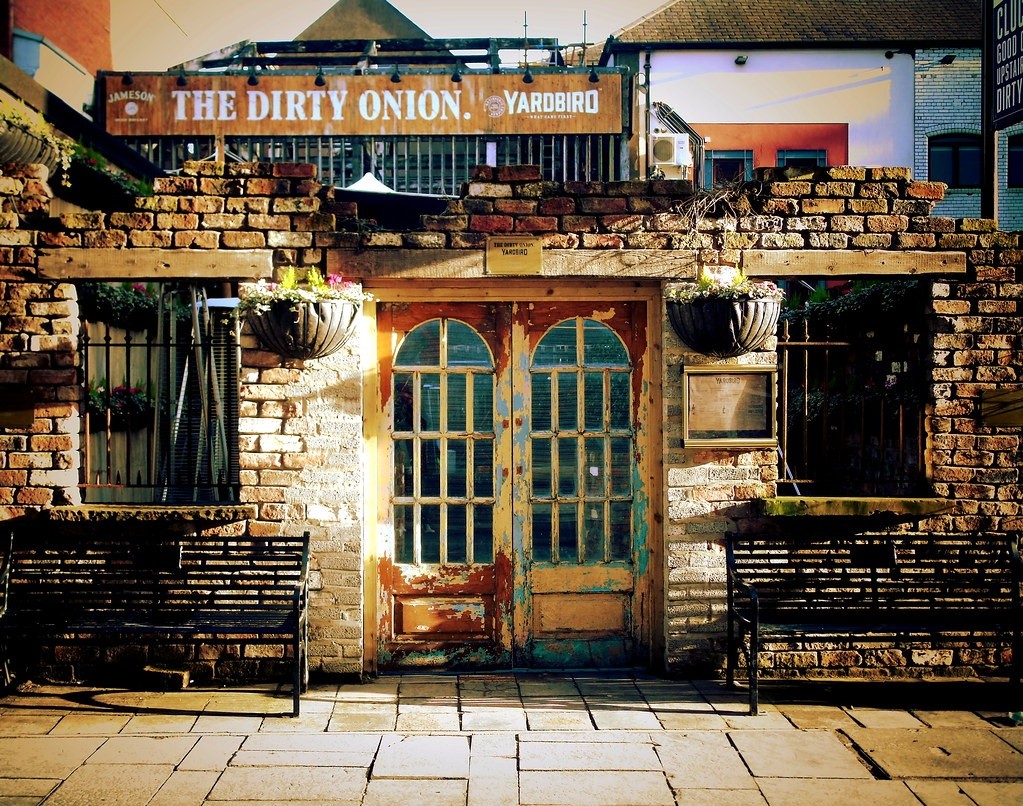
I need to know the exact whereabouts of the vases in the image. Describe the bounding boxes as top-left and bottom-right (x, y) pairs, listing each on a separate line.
(666, 295), (782, 357)
(89, 409), (154, 433)
(246, 300), (362, 358)
(87, 307), (192, 334)
(48, 158), (137, 214)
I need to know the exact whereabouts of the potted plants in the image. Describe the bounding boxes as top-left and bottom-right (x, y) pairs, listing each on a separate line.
(0, 98), (75, 188)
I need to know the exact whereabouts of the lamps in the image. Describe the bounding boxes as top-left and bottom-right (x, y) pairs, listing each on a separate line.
(315, 62), (326, 86)
(523, 64), (533, 83)
(177, 67), (186, 86)
(941, 55), (956, 64)
(451, 63), (462, 82)
(391, 62), (401, 82)
(248, 63), (259, 85)
(122, 71), (133, 84)
(636, 73), (649, 94)
(735, 56), (749, 64)
(589, 62), (600, 82)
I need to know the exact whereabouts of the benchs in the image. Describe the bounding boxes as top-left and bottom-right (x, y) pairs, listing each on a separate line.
(0, 531), (310, 718)
(724, 529), (1023, 717)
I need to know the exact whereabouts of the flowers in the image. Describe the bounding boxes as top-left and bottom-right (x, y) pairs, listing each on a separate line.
(87, 374), (159, 413)
(220, 264), (374, 337)
(664, 260), (786, 303)
(71, 135), (154, 197)
(79, 280), (203, 322)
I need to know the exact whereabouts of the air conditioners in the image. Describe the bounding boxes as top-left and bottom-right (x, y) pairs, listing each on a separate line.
(651, 133), (690, 166)
